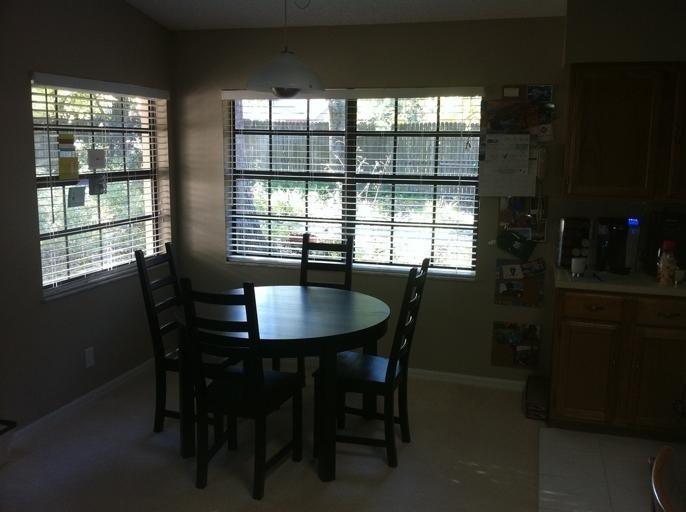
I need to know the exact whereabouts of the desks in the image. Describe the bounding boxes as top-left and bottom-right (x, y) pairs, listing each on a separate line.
(179, 285), (390, 482)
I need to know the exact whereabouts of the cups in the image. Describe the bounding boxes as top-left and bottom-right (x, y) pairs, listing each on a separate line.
(87, 148), (108, 170)
(59, 156), (80, 181)
(571, 256), (587, 283)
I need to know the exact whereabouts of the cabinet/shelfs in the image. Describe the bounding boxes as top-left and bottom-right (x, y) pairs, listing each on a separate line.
(549, 290), (686, 442)
(564, 60), (685, 205)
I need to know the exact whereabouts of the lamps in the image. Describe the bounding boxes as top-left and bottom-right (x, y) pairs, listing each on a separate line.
(247, 0), (326, 98)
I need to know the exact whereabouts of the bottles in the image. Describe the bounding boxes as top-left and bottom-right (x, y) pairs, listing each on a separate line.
(656, 239), (677, 285)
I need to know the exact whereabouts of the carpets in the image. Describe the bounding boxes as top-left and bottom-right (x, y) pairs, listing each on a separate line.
(1, 357), (546, 512)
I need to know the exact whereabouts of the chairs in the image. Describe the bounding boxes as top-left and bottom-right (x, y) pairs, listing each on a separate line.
(648, 445), (678, 512)
(176, 279), (301, 500)
(273, 233), (353, 386)
(313, 259), (429, 466)
(134, 243), (241, 443)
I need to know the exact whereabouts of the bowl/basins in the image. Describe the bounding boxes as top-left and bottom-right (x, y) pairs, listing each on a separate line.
(673, 269), (686, 283)
